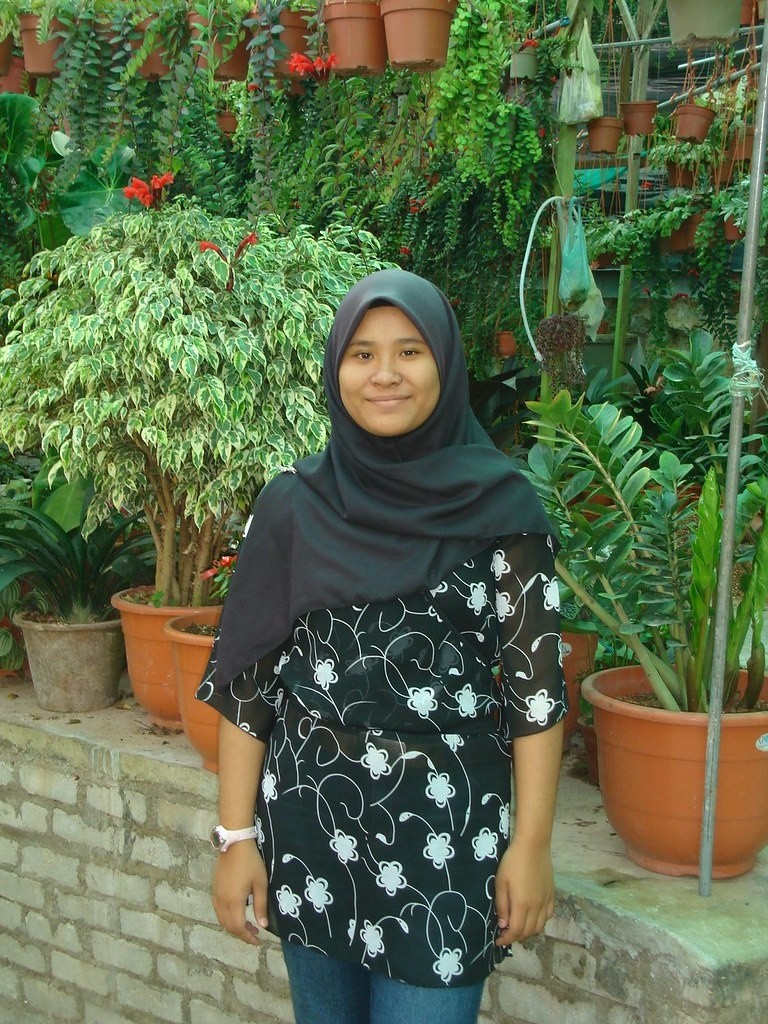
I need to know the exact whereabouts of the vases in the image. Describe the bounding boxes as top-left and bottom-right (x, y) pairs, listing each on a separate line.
(587, 117), (623, 155)
(159, 605), (223, 778)
(742, 0), (759, 27)
(666, 0), (743, 51)
(619, 100), (660, 134)
(674, 103), (717, 142)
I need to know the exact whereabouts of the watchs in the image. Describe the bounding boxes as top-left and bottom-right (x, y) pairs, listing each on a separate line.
(209, 824), (257, 852)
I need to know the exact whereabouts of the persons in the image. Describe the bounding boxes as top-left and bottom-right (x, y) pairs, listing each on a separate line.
(190, 267), (570, 1024)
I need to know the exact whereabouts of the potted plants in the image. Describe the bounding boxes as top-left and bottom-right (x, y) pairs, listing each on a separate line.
(0, 443), (181, 712)
(0, 192), (404, 732)
(493, 173), (768, 876)
(510, 33), (583, 105)
(705, 113), (734, 184)
(645, 140), (715, 188)
(0, 0), (460, 138)
(712, 73), (759, 161)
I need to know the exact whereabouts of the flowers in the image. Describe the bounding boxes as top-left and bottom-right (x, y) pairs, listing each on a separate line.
(191, 516), (251, 601)
